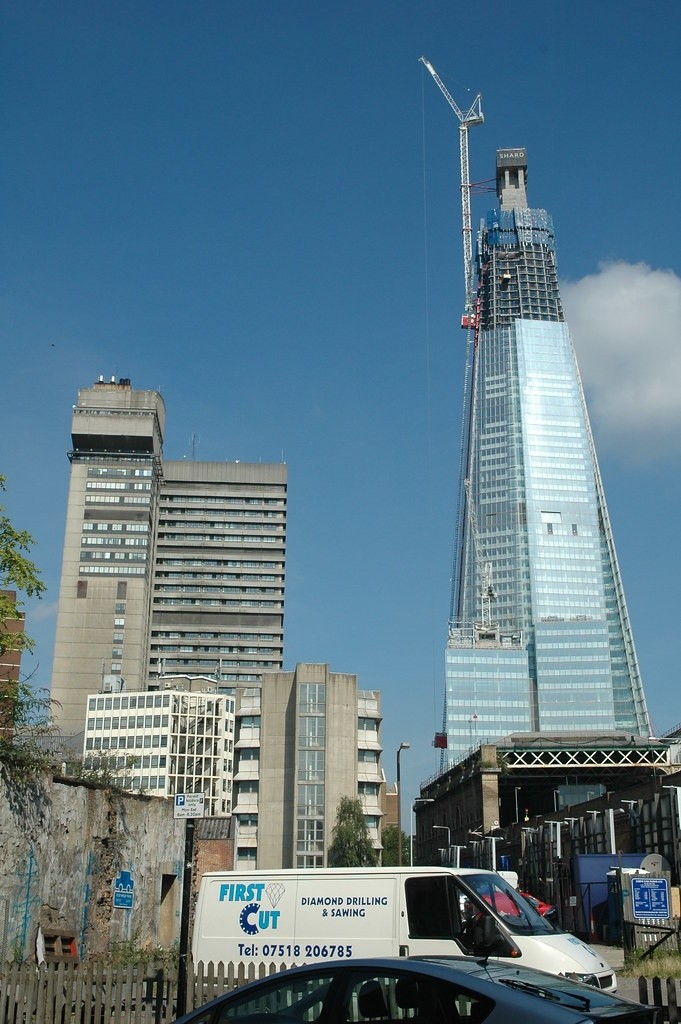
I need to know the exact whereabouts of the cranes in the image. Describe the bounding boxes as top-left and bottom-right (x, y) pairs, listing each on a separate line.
(460, 475), (501, 632)
(417, 52), (488, 331)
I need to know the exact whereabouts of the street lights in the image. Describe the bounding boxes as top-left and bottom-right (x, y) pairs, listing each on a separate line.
(553, 789), (560, 813)
(515, 786), (522, 824)
(396, 743), (411, 867)
(432, 825), (451, 866)
(588, 792), (594, 801)
(409, 799), (436, 867)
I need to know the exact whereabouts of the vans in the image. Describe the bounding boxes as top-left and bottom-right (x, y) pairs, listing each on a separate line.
(192, 866), (616, 998)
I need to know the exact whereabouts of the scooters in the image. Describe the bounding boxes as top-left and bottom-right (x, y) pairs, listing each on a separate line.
(459, 898), (557, 956)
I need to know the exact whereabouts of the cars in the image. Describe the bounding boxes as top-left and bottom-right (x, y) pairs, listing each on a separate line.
(167, 955), (680, 1024)
